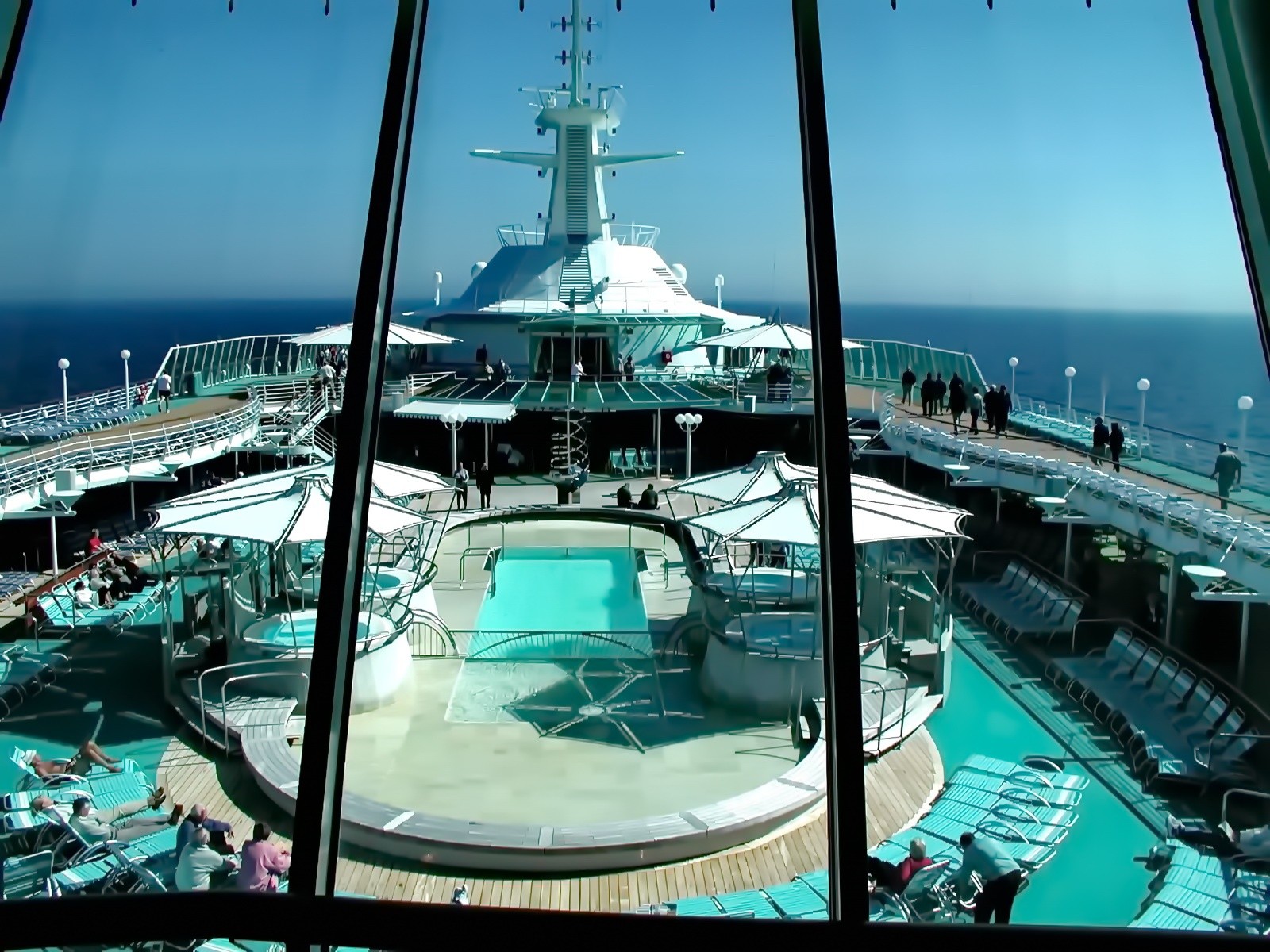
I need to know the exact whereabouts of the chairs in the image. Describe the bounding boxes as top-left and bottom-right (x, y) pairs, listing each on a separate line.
(610, 413), (1270, 935)
(0, 407), (374, 952)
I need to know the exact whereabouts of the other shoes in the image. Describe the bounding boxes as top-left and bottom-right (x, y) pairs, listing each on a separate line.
(458, 884), (471, 906)
(153, 786), (163, 798)
(452, 887), (462, 902)
(152, 795), (166, 811)
(168, 805), (183, 825)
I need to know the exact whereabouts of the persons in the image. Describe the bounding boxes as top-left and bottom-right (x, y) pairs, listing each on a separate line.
(21, 469), (290, 891)
(901, 366), (917, 407)
(1052, 550), (1074, 580)
(1108, 423), (1124, 472)
(159, 346), (791, 415)
(867, 814), (1270, 929)
(921, 373), (1012, 438)
(1091, 416), (1109, 467)
(454, 461), (789, 569)
(1078, 548), (1100, 620)
(1210, 443), (1242, 511)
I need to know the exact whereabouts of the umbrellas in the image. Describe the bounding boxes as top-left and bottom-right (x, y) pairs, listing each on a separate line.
(687, 321), (869, 381)
(282, 319), (463, 373)
(659, 448), (973, 655)
(144, 458), (465, 665)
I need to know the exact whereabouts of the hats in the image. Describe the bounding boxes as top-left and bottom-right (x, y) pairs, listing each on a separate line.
(21, 749), (36, 765)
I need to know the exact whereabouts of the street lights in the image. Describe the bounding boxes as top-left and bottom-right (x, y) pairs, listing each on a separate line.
(1136, 378), (1151, 461)
(1008, 357), (1019, 395)
(1237, 395), (1254, 462)
(57, 358), (70, 420)
(676, 412), (703, 481)
(121, 349), (132, 410)
(1065, 366), (1075, 421)
